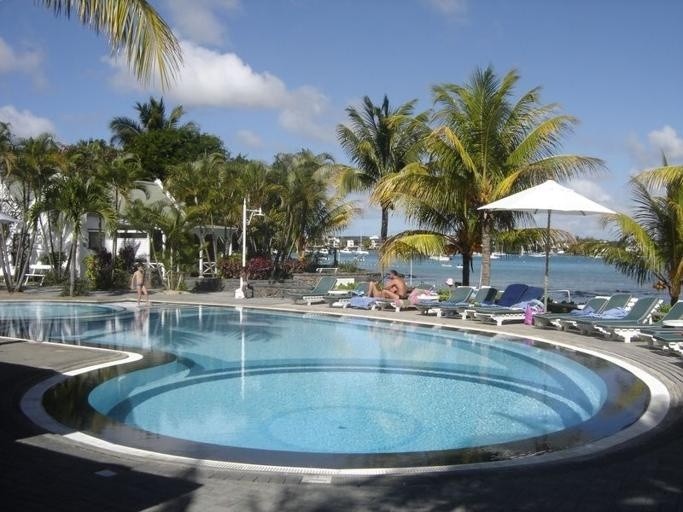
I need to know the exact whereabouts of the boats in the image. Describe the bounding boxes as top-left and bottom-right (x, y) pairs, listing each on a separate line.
(306, 238), (549, 270)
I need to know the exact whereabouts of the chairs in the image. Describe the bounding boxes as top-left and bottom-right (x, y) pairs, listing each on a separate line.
(532, 293), (683, 357)
(286, 276), (430, 312)
(413, 284), (545, 327)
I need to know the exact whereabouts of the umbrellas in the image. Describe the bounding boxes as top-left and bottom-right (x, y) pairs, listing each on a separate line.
(475, 179), (622, 314)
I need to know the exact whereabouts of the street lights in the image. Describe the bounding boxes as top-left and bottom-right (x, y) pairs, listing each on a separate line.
(234, 198), (265, 301)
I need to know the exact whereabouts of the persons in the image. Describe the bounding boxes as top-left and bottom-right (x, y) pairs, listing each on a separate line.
(129, 262), (149, 303)
(132, 306), (149, 340)
(380, 269), (408, 299)
(366, 280), (397, 298)
(238, 266), (255, 298)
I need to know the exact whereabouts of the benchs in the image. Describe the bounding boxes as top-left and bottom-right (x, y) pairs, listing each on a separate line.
(22, 264), (54, 287)
